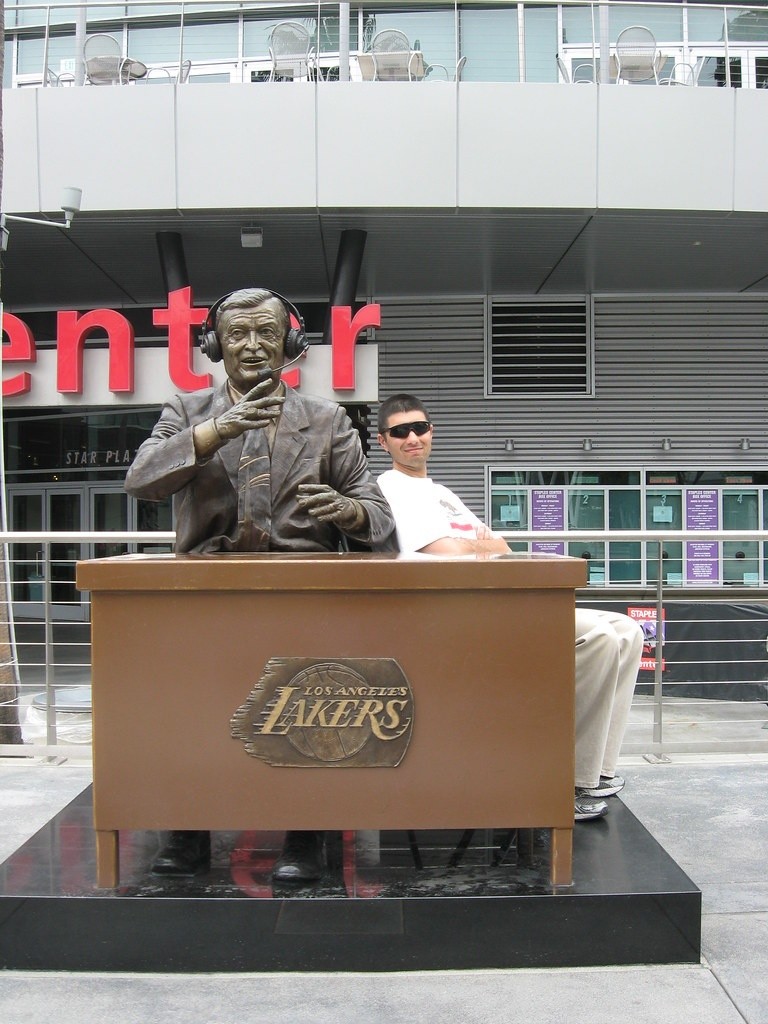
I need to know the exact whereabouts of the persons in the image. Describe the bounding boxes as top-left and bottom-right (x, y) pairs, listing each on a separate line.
(123, 287), (396, 882)
(375, 394), (643, 820)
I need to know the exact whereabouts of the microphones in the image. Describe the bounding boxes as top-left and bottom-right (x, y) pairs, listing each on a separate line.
(256, 349), (306, 380)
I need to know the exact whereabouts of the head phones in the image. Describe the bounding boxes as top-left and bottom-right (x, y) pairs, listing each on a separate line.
(198, 287), (309, 363)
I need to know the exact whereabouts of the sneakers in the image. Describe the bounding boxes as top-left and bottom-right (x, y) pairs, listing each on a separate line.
(580, 776), (625, 797)
(574, 787), (608, 820)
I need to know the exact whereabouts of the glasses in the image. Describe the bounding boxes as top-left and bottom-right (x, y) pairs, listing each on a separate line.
(380, 421), (431, 438)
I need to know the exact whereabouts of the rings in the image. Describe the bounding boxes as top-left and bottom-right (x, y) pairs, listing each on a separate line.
(248, 407), (259, 417)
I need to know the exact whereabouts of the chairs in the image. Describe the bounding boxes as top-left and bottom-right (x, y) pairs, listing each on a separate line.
(46, 21), (706, 87)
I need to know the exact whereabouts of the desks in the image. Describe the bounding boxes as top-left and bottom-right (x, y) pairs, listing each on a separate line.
(74, 552), (591, 887)
(609, 53), (667, 85)
(81, 55), (147, 85)
(356, 51), (424, 81)
